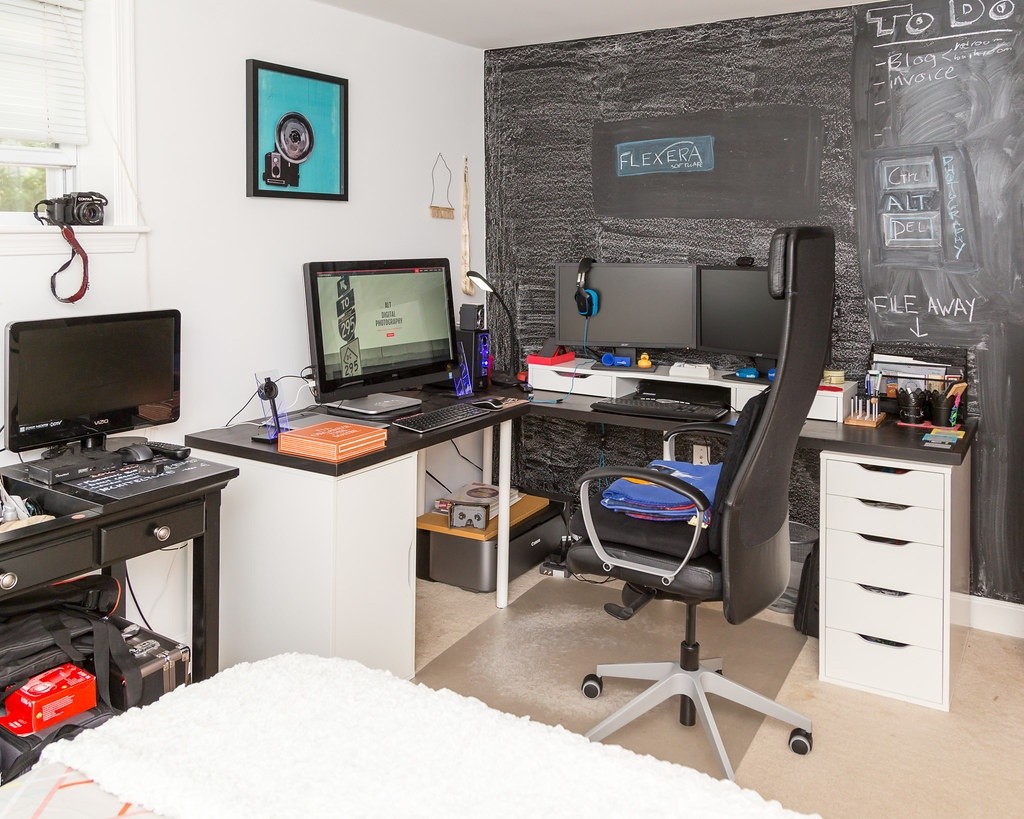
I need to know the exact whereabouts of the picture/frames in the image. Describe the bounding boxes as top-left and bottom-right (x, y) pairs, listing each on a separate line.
(247, 58), (351, 200)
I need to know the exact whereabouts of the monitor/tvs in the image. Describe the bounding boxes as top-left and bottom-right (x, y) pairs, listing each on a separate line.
(696, 265), (786, 386)
(3, 308), (181, 455)
(554, 262), (696, 373)
(303, 257), (458, 420)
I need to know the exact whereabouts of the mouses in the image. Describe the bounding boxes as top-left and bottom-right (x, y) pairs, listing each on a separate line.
(472, 398), (503, 410)
(114, 444), (153, 464)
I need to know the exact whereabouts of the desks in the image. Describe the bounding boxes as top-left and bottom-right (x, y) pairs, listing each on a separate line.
(0, 434), (243, 680)
(186, 371), (989, 502)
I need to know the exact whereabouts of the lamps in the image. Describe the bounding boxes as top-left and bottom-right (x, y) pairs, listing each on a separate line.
(467, 269), (526, 388)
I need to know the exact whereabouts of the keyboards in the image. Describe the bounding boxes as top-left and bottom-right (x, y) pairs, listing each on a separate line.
(590, 397), (729, 422)
(391, 402), (490, 433)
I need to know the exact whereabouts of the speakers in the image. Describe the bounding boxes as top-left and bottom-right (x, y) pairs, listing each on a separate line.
(459, 303), (484, 331)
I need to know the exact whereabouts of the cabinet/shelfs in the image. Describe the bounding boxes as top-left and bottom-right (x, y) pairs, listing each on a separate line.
(816, 449), (977, 711)
(179, 446), (422, 681)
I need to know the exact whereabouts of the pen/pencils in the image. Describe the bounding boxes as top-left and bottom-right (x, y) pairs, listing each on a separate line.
(864, 372), (871, 395)
(875, 370), (883, 395)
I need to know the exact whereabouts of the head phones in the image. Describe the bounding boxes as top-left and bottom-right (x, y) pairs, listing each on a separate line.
(574, 258), (599, 317)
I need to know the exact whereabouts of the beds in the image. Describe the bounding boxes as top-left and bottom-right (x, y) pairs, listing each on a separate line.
(1, 652), (821, 817)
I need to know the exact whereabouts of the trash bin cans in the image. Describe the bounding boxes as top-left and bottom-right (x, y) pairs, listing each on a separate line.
(766, 520), (819, 616)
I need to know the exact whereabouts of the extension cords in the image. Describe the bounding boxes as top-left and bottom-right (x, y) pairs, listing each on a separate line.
(539, 560), (572, 578)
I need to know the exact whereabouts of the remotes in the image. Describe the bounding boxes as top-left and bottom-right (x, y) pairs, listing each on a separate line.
(141, 441), (191, 460)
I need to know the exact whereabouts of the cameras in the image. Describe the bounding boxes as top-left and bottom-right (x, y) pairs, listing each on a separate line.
(34, 191), (108, 226)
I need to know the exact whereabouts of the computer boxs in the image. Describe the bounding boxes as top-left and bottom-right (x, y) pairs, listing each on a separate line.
(422, 326), (490, 393)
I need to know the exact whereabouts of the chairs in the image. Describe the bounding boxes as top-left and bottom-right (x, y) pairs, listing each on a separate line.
(564, 224), (837, 783)
